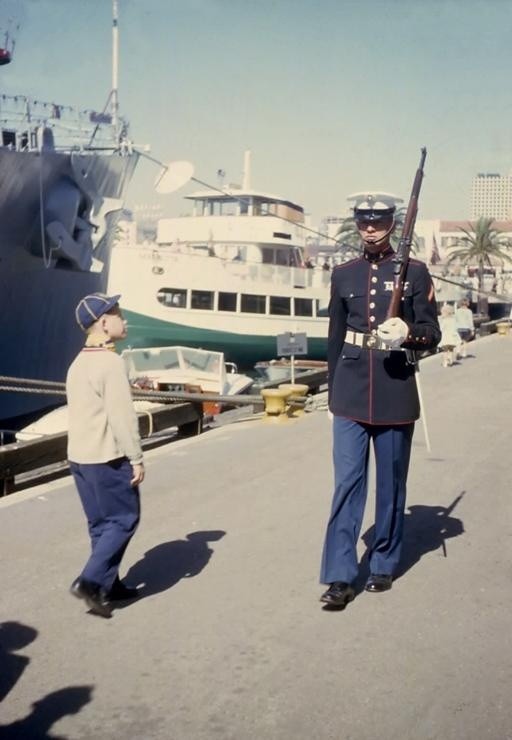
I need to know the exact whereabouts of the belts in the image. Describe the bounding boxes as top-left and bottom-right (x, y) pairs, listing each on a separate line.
(344, 330), (406, 351)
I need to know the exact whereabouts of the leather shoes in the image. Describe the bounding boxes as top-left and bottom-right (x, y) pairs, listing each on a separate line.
(72, 576), (137, 618)
(320, 581), (355, 605)
(366, 573), (392, 591)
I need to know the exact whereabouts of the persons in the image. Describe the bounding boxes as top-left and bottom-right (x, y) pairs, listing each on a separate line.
(454, 298), (475, 359)
(317, 191), (444, 608)
(64, 291), (146, 620)
(437, 304), (462, 367)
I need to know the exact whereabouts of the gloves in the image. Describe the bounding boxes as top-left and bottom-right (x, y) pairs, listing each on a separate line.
(377, 318), (409, 346)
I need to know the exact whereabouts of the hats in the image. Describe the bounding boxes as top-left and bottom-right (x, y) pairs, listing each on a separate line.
(75, 293), (120, 331)
(354, 199), (396, 221)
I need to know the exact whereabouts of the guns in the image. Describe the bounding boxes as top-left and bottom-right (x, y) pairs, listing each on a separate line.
(386, 147), (426, 320)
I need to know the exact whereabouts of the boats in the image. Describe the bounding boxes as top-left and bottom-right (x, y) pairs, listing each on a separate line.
(1, 1), (140, 447)
(14, 345), (254, 445)
(427, 270), (511, 339)
(254, 357), (328, 382)
(108, 150), (330, 393)
